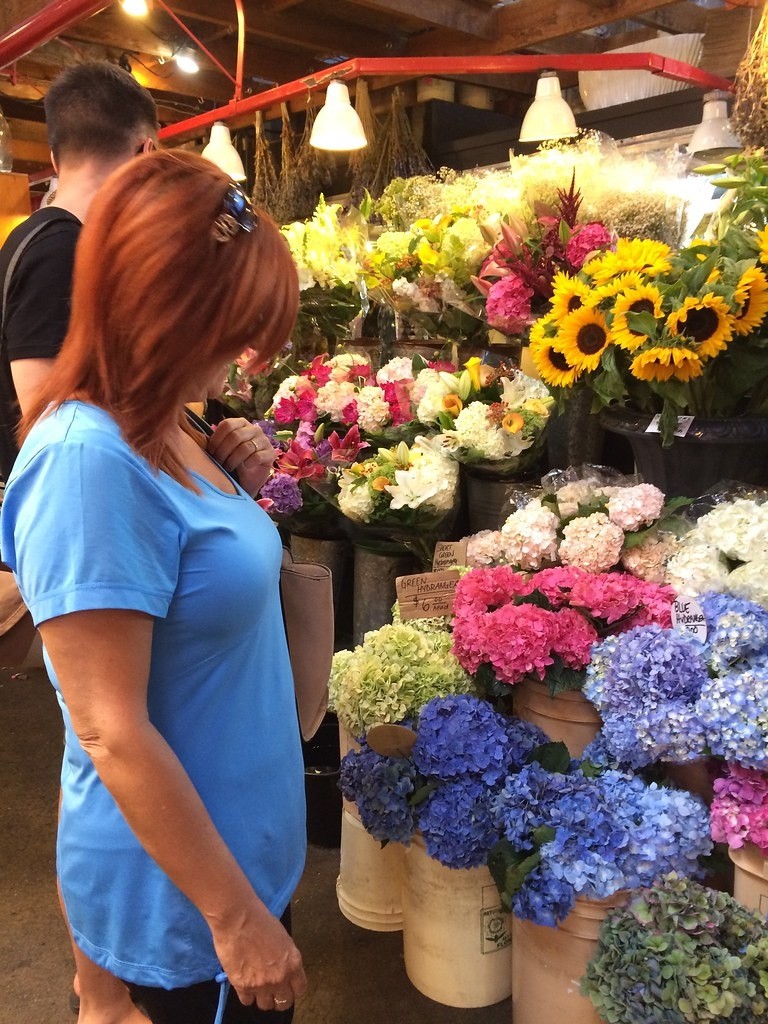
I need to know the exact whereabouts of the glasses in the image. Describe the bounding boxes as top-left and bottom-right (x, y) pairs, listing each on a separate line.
(210, 180), (261, 248)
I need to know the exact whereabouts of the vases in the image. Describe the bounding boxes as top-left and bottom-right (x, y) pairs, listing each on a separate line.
(346, 333), (386, 369)
(727, 839), (768, 915)
(666, 750), (723, 804)
(334, 805), (402, 935)
(337, 700), (380, 824)
(390, 338), (455, 363)
(347, 537), (417, 651)
(504, 883), (643, 1024)
(399, 827), (511, 1009)
(465, 455), (519, 532)
(597, 397), (768, 497)
(291, 525), (343, 581)
(508, 677), (600, 762)
(458, 346), (509, 370)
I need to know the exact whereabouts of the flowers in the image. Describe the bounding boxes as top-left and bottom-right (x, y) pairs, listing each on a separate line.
(213, 127), (768, 1024)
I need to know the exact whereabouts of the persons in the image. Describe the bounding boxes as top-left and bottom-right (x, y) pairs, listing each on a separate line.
(0, 148), (310, 1024)
(0, 59), (207, 1024)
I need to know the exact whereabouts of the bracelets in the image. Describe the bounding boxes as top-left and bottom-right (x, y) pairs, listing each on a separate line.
(203, 400), (208, 413)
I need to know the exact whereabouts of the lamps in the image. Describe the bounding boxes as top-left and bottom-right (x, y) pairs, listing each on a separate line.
(175, 39), (202, 75)
(202, 116), (249, 186)
(516, 68), (580, 144)
(305, 81), (370, 156)
(685, 87), (747, 162)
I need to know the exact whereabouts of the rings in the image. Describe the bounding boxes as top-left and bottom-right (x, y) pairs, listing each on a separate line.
(275, 997), (287, 1004)
(251, 440), (258, 451)
